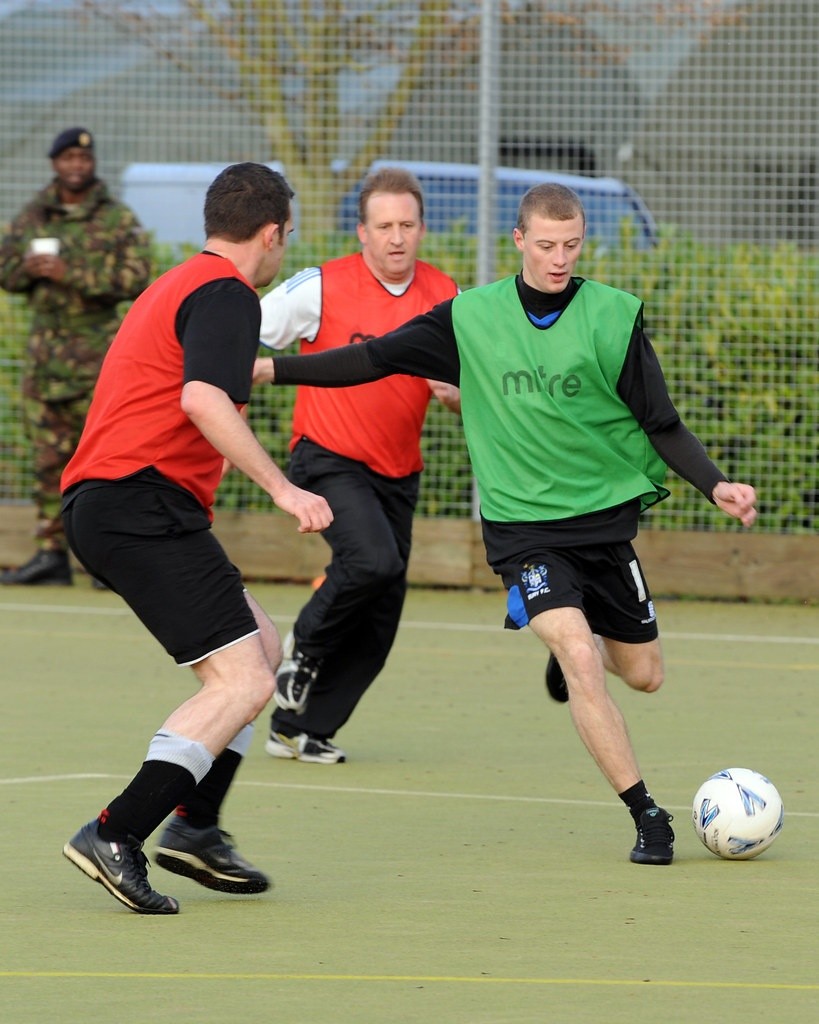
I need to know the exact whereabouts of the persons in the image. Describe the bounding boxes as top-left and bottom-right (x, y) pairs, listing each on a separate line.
(60, 162), (335, 912)
(0, 128), (151, 594)
(252, 181), (759, 863)
(260, 170), (461, 765)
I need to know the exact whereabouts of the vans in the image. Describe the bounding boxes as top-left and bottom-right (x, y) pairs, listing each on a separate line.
(120, 158), (659, 254)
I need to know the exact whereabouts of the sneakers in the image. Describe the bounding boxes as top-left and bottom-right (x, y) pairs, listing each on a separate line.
(62, 818), (178, 914)
(546, 651), (571, 702)
(266, 726), (345, 764)
(155, 815), (269, 894)
(629, 808), (676, 863)
(272, 628), (319, 710)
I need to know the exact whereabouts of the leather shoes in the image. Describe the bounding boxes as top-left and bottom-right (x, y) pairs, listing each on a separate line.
(0, 548), (73, 587)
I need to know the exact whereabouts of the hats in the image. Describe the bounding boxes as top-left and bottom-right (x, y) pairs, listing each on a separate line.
(49, 127), (92, 159)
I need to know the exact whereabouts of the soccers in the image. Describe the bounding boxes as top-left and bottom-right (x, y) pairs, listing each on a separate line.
(691, 767), (785, 862)
